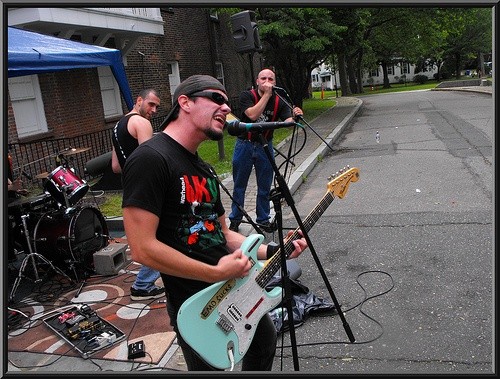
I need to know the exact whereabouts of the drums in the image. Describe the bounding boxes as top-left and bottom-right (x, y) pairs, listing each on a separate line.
(42, 164), (90, 207)
(30, 203), (110, 270)
(31, 191), (57, 211)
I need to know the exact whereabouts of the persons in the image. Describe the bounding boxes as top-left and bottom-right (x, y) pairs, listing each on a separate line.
(229, 68), (302, 233)
(112, 88), (165, 301)
(121, 75), (277, 371)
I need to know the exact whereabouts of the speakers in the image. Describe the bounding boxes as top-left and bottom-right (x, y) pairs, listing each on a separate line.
(230, 10), (262, 52)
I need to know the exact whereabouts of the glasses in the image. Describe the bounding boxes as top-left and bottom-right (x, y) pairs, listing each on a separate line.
(188, 91), (229, 106)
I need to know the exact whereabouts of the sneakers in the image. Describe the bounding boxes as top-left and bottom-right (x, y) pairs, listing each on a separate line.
(130, 285), (166, 301)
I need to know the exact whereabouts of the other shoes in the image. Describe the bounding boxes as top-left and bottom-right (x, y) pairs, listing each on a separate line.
(261, 223), (272, 233)
(229, 223), (239, 232)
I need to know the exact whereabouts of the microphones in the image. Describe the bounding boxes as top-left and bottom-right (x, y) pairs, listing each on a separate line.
(271, 85), (285, 91)
(228, 121), (296, 136)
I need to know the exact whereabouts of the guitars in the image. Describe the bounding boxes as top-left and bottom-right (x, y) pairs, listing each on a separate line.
(176, 164), (359, 369)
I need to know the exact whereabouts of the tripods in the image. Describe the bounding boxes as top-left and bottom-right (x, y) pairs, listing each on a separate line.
(6, 206), (73, 303)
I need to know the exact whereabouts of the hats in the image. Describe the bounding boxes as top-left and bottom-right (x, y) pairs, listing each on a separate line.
(158, 74), (227, 130)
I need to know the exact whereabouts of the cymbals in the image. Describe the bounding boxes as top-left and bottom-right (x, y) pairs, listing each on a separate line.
(35, 167), (75, 179)
(7, 193), (47, 207)
(50, 146), (92, 158)
